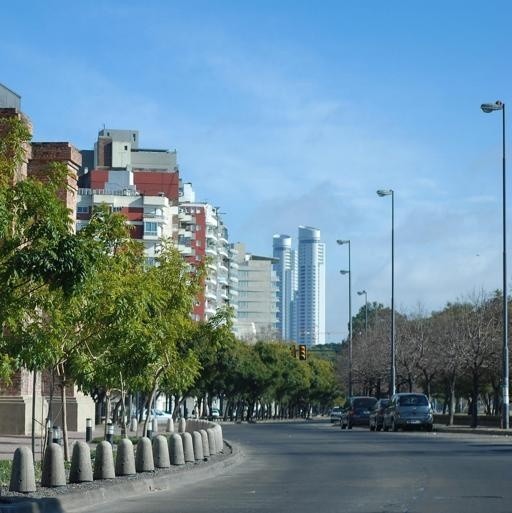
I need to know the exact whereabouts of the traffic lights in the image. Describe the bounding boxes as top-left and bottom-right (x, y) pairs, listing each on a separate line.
(299, 344), (307, 360)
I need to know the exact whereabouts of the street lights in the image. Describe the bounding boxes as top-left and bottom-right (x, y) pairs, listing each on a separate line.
(339, 269), (351, 274)
(375, 188), (396, 398)
(335, 239), (353, 398)
(481, 97), (512, 428)
(355, 289), (368, 343)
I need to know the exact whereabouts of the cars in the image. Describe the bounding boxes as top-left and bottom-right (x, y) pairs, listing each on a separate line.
(126, 405), (269, 421)
(330, 392), (433, 431)
